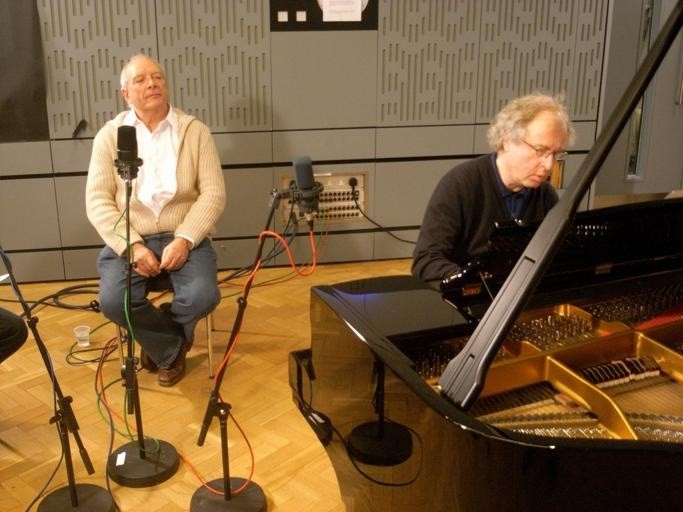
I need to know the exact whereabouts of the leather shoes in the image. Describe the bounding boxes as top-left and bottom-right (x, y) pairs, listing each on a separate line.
(141, 346), (157, 370)
(158, 334), (195, 387)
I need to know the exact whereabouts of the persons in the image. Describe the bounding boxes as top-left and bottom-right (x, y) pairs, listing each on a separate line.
(411, 94), (576, 282)
(86, 54), (226, 387)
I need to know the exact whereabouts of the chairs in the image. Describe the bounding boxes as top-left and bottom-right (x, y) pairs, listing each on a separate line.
(117, 270), (216, 379)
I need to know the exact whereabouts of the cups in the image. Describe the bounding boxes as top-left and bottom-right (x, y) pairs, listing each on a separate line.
(73, 325), (90, 347)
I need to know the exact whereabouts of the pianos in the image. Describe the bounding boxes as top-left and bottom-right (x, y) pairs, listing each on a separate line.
(289, 194), (681, 512)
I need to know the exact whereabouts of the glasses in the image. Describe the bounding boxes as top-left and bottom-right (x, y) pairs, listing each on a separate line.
(520, 138), (568, 161)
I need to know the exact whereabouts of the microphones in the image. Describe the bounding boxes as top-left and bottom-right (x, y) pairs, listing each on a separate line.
(292, 156), (320, 231)
(114, 125), (144, 197)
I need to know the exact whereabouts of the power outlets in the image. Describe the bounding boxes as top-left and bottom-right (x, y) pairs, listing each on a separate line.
(283, 174), (363, 191)
